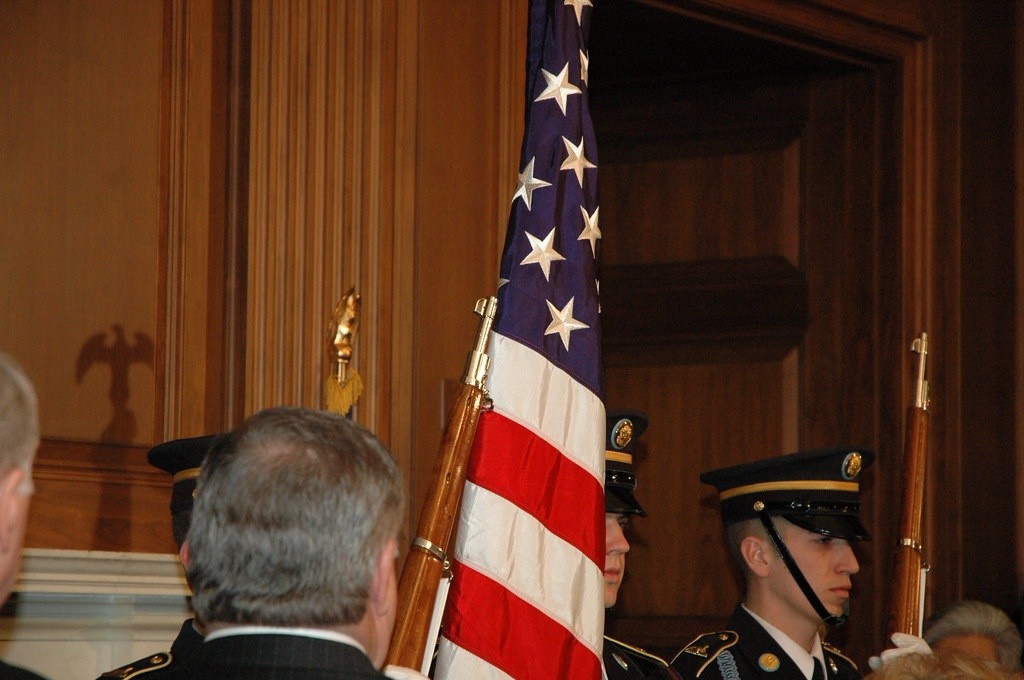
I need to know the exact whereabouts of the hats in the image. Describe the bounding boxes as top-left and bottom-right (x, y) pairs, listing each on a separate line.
(699, 446), (874, 541)
(604, 410), (647, 516)
(146, 433), (225, 510)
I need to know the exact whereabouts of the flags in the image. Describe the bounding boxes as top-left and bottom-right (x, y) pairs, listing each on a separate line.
(431, 1), (607, 680)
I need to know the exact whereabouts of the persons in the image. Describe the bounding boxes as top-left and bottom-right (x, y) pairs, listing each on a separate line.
(180, 406), (405, 680)
(926, 600), (1024, 672)
(97, 433), (215, 680)
(669, 445), (933, 680)
(0, 352), (49, 680)
(866, 652), (1020, 680)
(606, 412), (680, 680)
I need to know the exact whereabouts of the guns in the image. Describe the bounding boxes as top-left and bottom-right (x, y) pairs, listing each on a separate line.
(377, 293), (501, 679)
(889, 331), (931, 648)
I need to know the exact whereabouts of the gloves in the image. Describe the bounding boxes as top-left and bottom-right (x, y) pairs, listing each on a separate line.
(868, 633), (933, 671)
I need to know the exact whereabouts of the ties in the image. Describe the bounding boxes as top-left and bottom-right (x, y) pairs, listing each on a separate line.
(811, 657), (824, 680)
